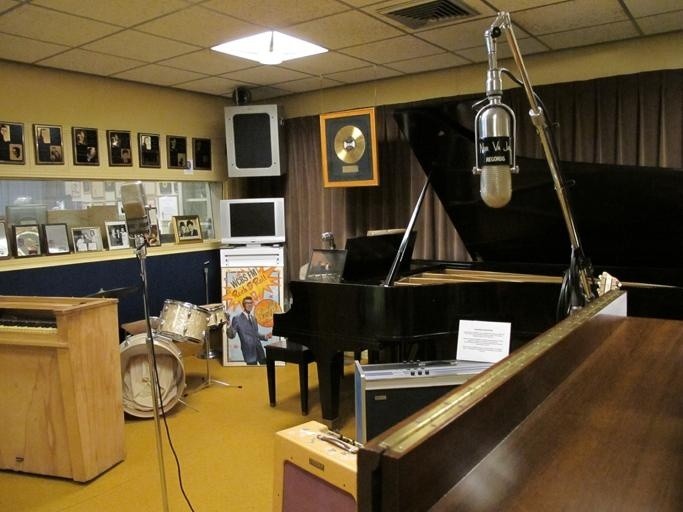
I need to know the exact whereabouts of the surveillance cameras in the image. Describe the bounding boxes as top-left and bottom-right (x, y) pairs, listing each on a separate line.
(232, 88), (252, 105)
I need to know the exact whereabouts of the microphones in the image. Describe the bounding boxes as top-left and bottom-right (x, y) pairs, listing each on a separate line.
(121, 181), (151, 235)
(472, 68), (520, 210)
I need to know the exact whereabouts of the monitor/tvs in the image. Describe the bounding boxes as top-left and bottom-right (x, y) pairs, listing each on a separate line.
(220, 198), (286, 248)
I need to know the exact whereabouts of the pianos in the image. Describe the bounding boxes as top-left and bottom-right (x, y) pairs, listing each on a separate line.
(0, 311), (59, 336)
(268, 104), (681, 421)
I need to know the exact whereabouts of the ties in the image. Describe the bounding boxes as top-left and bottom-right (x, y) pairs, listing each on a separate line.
(248, 315), (252, 325)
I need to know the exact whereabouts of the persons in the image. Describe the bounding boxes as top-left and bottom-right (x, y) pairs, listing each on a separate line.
(18, 238), (29, 255)
(52, 147), (60, 161)
(77, 132), (85, 144)
(180, 222), (188, 237)
(120, 227), (127, 245)
(87, 147), (96, 162)
(109, 227), (117, 246)
(179, 158), (184, 167)
(12, 148), (20, 158)
(1, 126), (8, 141)
(40, 129), (48, 142)
(226, 297), (274, 366)
(170, 144), (175, 152)
(89, 230), (98, 250)
(76, 234), (88, 252)
(113, 134), (120, 147)
(186, 221), (198, 236)
(142, 136), (149, 150)
(115, 228), (122, 245)
(122, 150), (129, 162)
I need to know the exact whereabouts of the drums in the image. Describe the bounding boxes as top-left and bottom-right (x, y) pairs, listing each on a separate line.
(120, 333), (184, 418)
(154, 298), (212, 348)
(197, 303), (232, 328)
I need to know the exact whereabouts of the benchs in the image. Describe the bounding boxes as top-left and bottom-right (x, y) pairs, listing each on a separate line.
(267, 333), (349, 414)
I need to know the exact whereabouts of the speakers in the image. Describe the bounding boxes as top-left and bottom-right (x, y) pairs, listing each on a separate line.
(224, 104), (280, 177)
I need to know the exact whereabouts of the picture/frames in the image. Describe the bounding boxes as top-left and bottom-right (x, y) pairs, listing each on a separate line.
(44, 222), (70, 254)
(105, 222), (134, 250)
(168, 135), (188, 168)
(0, 120), (24, 164)
(173, 214), (203, 244)
(34, 124), (64, 164)
(320, 106), (380, 188)
(71, 127), (98, 164)
(0, 221), (11, 259)
(136, 132), (161, 169)
(72, 227), (102, 250)
(191, 137), (211, 170)
(13, 224), (42, 256)
(304, 248), (348, 285)
(221, 267), (286, 367)
(105, 130), (132, 166)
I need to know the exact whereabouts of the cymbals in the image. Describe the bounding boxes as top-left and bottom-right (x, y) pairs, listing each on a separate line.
(86, 286), (139, 298)
